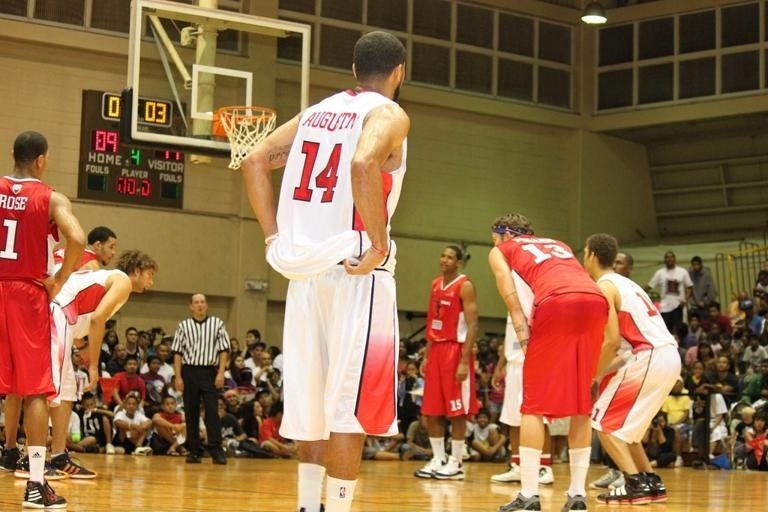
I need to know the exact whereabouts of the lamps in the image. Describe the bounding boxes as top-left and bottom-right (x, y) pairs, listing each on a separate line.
(581, 0), (607, 24)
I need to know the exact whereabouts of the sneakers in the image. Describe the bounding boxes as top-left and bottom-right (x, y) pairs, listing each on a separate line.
(414, 455), (448, 478)
(21, 479), (69, 510)
(488, 462), (522, 484)
(671, 455), (685, 469)
(72, 436), (300, 459)
(397, 444), (418, 460)
(539, 464), (554, 485)
(589, 468), (669, 506)
(0, 445), (22, 472)
(47, 448), (98, 479)
(13, 455), (69, 481)
(560, 492), (588, 512)
(433, 456), (466, 481)
(496, 492), (542, 512)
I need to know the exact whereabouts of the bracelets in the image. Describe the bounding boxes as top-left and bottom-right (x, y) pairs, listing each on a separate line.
(263, 231), (279, 244)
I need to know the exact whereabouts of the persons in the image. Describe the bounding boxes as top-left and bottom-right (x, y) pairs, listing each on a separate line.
(488, 212), (611, 512)
(1, 131), (86, 509)
(53, 224), (118, 272)
(170, 292), (233, 466)
(363, 245), (768, 489)
(240, 29), (412, 512)
(13, 249), (158, 482)
(0, 318), (299, 457)
(581, 230), (684, 505)
